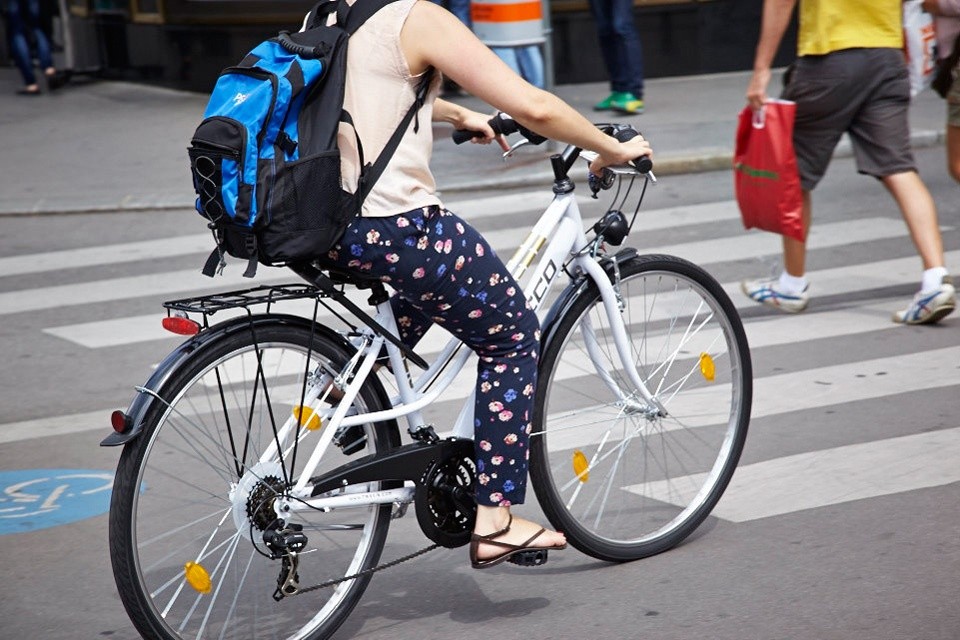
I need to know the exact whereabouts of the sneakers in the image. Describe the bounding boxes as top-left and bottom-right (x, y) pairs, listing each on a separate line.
(739, 278), (807, 315)
(609, 94), (644, 114)
(595, 91), (623, 110)
(893, 287), (953, 325)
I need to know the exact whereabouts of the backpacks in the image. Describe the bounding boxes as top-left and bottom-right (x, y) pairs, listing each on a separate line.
(189, 0), (438, 279)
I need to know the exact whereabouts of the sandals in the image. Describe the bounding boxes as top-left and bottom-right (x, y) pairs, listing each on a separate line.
(469, 513), (567, 569)
(313, 388), (356, 407)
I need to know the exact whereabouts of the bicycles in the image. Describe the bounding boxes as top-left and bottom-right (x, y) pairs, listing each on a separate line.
(96, 107), (757, 639)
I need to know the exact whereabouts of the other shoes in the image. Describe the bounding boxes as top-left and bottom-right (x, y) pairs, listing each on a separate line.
(40, 68), (59, 82)
(17, 87), (40, 94)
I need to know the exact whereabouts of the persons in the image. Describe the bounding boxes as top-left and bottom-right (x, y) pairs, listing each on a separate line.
(740, 0), (957, 326)
(0, 0), (69, 97)
(298, 0), (657, 570)
(920, 0), (960, 184)
(586, 0), (646, 115)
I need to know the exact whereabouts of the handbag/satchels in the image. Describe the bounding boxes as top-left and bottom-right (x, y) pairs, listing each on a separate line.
(732, 98), (806, 242)
(898, 0), (936, 95)
